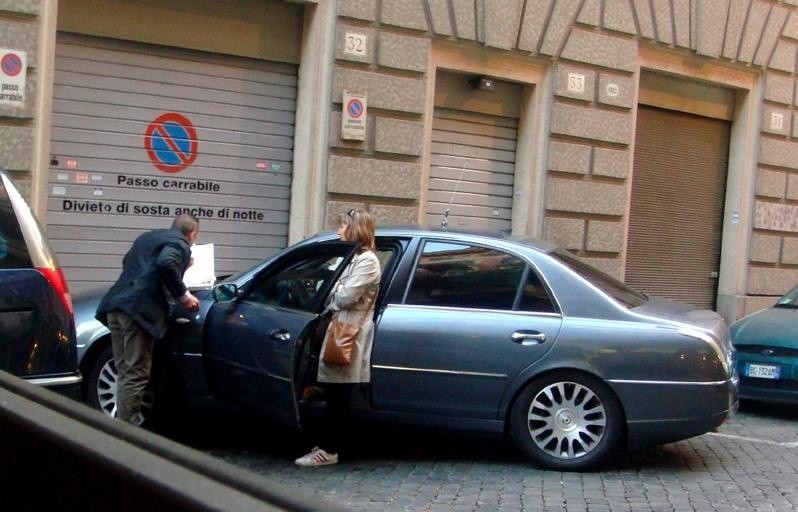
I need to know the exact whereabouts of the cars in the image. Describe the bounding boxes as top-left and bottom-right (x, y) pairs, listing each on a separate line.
(69, 225), (740, 460)
(727, 282), (798, 406)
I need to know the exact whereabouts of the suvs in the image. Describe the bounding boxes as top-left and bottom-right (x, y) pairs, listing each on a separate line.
(0, 168), (82, 392)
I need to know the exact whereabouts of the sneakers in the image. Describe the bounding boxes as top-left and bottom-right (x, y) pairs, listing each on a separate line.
(295, 446), (338, 466)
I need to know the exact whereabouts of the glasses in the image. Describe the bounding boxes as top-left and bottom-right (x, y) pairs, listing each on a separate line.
(347, 209), (354, 220)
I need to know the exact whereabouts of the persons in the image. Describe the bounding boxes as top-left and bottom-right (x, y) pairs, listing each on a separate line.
(293, 207), (382, 467)
(94, 213), (200, 428)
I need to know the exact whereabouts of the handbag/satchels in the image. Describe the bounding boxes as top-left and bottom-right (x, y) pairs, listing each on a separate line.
(322, 320), (360, 366)
